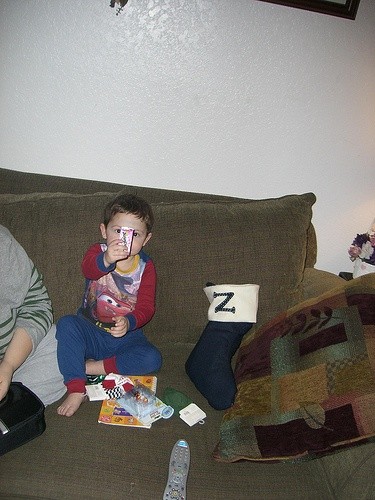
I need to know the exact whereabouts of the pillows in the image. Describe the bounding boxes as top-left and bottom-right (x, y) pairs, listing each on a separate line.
(0, 185), (140, 320)
(213, 272), (375, 463)
(142, 193), (317, 350)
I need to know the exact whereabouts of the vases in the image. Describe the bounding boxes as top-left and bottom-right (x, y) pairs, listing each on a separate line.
(353, 258), (375, 279)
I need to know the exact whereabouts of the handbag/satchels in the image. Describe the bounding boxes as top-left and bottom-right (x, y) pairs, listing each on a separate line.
(0, 380), (46, 456)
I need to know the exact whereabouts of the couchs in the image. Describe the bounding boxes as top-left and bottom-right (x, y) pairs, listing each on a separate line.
(0, 166), (375, 500)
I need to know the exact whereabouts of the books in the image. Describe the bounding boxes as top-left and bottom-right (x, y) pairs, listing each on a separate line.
(86, 373), (174, 430)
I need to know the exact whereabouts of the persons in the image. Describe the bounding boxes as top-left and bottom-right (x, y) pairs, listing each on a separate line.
(54, 194), (163, 418)
(0, 224), (67, 410)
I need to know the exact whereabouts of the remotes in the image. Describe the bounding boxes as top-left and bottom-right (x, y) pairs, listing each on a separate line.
(163, 438), (191, 500)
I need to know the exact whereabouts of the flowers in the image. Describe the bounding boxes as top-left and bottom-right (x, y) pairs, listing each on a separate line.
(347, 220), (375, 266)
